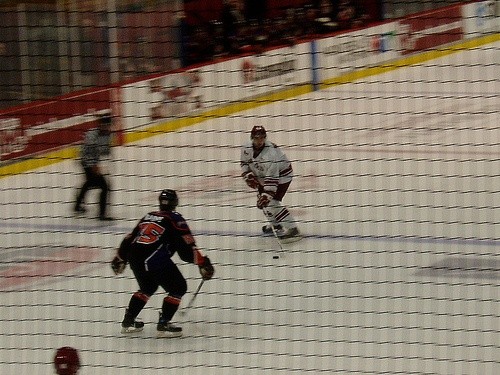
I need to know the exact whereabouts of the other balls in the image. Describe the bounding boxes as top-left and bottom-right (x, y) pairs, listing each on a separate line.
(272, 255), (279, 259)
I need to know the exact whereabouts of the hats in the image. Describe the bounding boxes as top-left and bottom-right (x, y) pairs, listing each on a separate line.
(250, 125), (266, 138)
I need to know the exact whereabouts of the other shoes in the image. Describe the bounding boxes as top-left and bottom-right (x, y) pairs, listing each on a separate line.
(74, 205), (86, 213)
(99, 214), (114, 222)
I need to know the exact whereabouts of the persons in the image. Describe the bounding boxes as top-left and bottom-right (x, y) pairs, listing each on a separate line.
(178, 0), (377, 67)
(73, 115), (117, 222)
(241, 124), (303, 243)
(111, 189), (214, 338)
(52, 347), (81, 375)
(0, 0), (180, 110)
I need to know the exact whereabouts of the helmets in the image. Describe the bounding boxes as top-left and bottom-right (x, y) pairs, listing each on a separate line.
(159, 189), (178, 211)
(53, 346), (79, 374)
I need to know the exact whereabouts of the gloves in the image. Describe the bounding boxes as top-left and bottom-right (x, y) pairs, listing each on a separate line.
(110, 252), (127, 275)
(198, 256), (214, 280)
(242, 170), (258, 190)
(256, 191), (275, 209)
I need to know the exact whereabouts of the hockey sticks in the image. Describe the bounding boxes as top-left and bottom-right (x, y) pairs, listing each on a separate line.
(255, 184), (285, 253)
(188, 278), (206, 307)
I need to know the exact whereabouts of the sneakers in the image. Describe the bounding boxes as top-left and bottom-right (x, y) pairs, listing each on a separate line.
(121, 308), (144, 333)
(155, 311), (182, 338)
(279, 228), (304, 244)
(262, 224), (283, 236)
